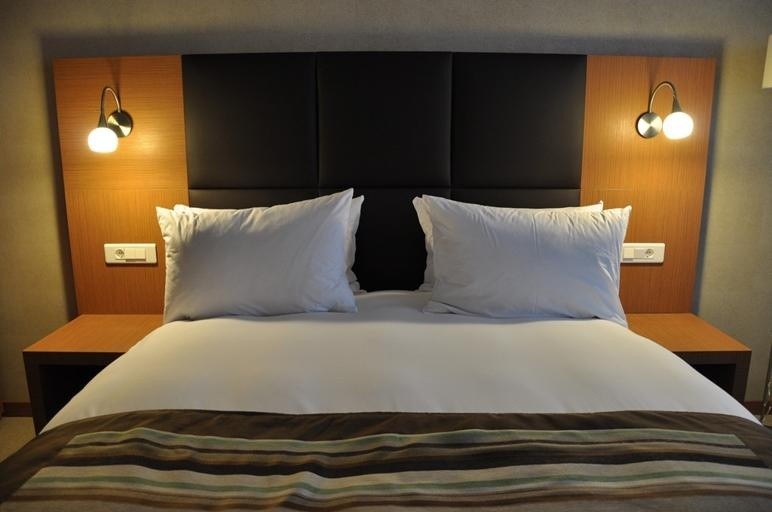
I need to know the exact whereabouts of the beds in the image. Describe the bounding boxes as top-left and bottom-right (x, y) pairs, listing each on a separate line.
(0, 290), (772, 512)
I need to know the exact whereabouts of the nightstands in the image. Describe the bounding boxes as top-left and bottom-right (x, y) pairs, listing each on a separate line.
(625, 311), (752, 406)
(21, 314), (166, 433)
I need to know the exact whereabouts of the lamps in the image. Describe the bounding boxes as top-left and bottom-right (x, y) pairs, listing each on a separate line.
(87, 86), (132, 153)
(635, 82), (693, 140)
